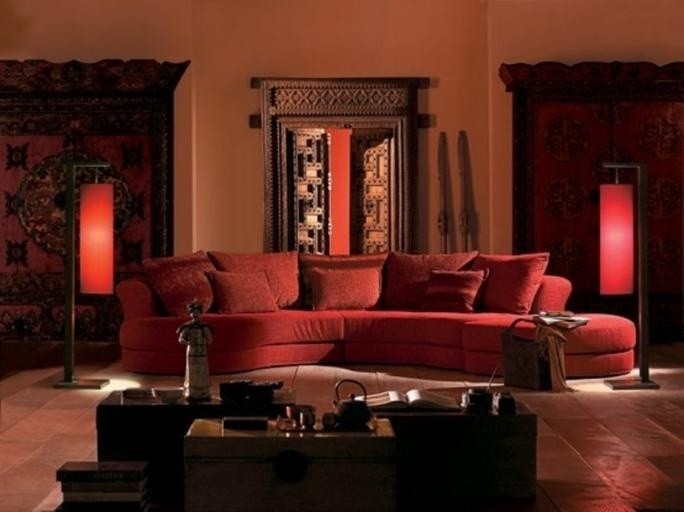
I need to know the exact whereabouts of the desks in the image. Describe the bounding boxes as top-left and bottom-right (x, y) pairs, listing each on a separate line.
(97, 383), (536, 502)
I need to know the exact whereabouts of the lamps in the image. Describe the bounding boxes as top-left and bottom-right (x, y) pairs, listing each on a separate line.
(50, 157), (116, 391)
(598, 155), (658, 391)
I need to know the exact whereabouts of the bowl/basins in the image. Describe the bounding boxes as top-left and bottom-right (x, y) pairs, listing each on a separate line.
(151, 387), (185, 404)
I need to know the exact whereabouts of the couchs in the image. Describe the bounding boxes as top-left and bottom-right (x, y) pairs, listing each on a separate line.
(110, 250), (637, 376)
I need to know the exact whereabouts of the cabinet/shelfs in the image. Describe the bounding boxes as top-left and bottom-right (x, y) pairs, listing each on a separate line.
(0, 61), (189, 343)
(497, 62), (684, 347)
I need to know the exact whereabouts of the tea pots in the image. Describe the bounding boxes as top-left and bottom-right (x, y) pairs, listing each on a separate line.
(332, 379), (372, 426)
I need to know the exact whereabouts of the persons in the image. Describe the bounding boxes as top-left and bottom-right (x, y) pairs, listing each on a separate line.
(176, 296), (213, 400)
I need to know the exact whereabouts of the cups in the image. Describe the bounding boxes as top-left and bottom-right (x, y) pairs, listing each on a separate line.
(323, 412), (337, 428)
(300, 412), (316, 429)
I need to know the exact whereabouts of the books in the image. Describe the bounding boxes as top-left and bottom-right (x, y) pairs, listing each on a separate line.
(534, 313), (588, 330)
(354, 389), (462, 413)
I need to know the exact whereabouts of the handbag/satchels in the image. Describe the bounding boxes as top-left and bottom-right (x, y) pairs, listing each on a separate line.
(501, 317), (567, 392)
